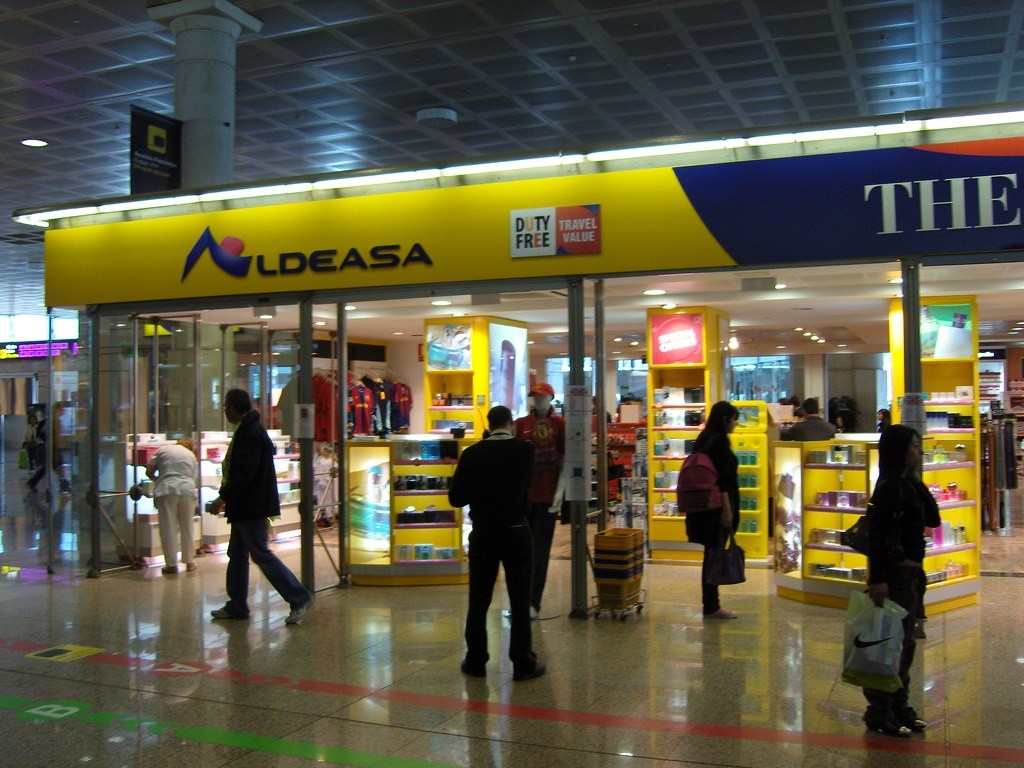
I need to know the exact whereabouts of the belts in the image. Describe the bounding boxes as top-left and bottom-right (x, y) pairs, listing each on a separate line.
(983, 419), (1006, 532)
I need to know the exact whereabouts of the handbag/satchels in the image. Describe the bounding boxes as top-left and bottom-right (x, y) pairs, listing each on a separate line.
(848, 478), (905, 564)
(705, 525), (747, 585)
(841, 590), (908, 693)
(18, 448), (28, 469)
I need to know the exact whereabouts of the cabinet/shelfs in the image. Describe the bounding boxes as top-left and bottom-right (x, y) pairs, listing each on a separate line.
(346, 372), (490, 587)
(773, 359), (1024, 616)
(646, 364), (769, 562)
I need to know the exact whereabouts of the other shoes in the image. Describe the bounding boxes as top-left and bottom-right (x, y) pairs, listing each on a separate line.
(61, 486), (71, 492)
(512, 661), (545, 681)
(187, 562), (198, 571)
(461, 652), (486, 676)
(26, 480), (38, 493)
(162, 566), (178, 573)
(892, 705), (927, 731)
(862, 707), (911, 738)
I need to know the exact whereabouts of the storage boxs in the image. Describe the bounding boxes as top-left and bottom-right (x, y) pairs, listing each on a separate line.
(594, 528), (644, 609)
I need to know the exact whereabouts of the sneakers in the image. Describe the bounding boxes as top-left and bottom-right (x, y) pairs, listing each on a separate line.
(286, 596), (313, 624)
(211, 607), (232, 619)
(704, 608), (737, 618)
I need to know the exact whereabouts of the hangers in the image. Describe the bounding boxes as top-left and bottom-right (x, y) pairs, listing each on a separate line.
(349, 368), (406, 387)
(314, 368), (338, 386)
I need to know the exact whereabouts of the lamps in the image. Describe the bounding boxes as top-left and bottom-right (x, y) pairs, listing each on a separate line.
(416, 108), (457, 129)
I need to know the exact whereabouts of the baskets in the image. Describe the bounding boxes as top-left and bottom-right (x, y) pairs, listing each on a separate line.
(594, 528), (644, 610)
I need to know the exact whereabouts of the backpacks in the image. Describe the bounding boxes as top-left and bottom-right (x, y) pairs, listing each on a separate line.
(677, 435), (722, 512)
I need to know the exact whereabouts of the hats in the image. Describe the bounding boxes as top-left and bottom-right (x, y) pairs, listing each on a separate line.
(528, 383), (554, 397)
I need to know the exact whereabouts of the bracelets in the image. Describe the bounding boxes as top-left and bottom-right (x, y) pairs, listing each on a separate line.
(838, 426), (843, 429)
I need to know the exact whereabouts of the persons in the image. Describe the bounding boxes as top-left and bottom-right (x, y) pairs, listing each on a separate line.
(27, 405), (70, 491)
(828, 400), (846, 431)
(448, 406), (543, 684)
(789, 396), (804, 418)
(781, 398), (839, 440)
(592, 396), (611, 423)
(146, 437), (198, 572)
(211, 389), (314, 623)
(22, 415), (39, 470)
(864, 424), (941, 736)
(513, 384), (565, 618)
(877, 409), (891, 432)
(677, 401), (741, 617)
